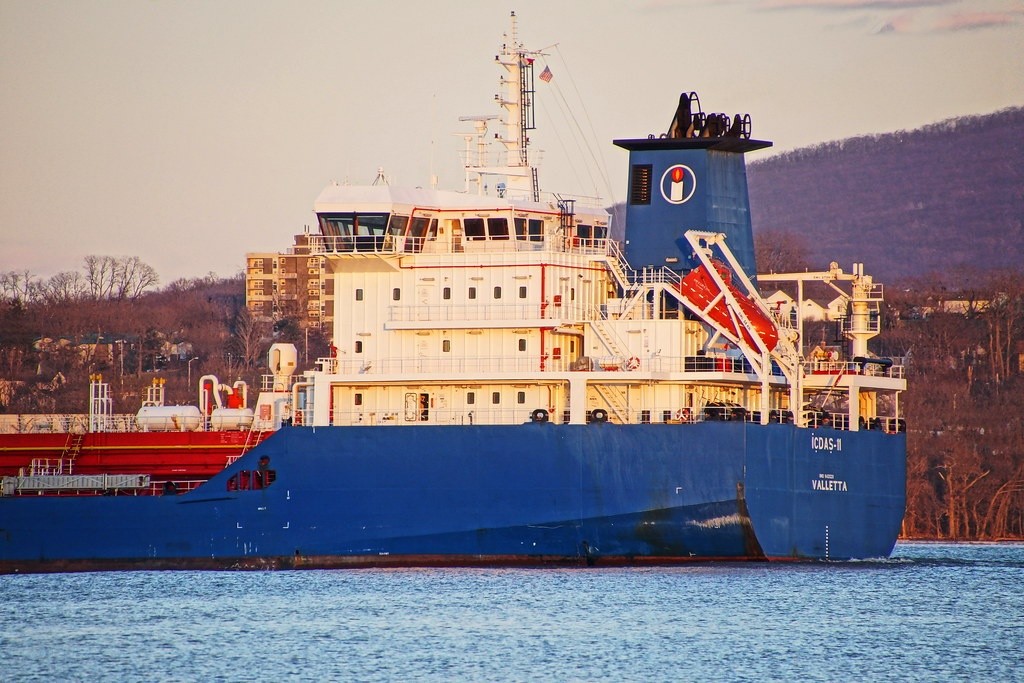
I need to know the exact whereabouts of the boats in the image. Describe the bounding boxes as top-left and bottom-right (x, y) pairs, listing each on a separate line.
(671, 258), (780, 354)
(0, 7), (910, 574)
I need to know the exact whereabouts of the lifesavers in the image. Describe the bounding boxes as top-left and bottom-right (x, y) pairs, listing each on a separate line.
(590, 409), (608, 423)
(628, 357), (640, 369)
(859, 416), (881, 431)
(163, 481), (179, 495)
(532, 409), (548, 424)
(890, 419), (906, 432)
(677, 409), (689, 422)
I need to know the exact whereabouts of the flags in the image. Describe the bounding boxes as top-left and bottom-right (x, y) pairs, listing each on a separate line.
(539, 66), (553, 82)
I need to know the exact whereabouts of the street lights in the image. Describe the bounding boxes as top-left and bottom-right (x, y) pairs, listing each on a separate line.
(227, 353), (232, 387)
(115, 339), (128, 389)
(187, 357), (198, 392)
